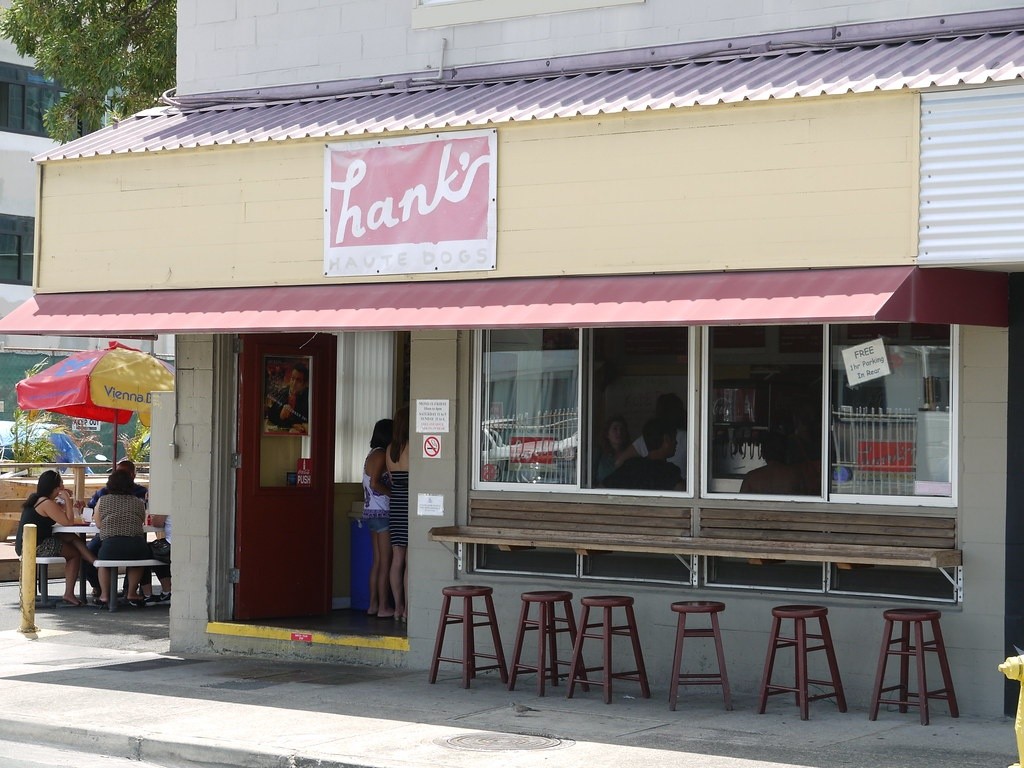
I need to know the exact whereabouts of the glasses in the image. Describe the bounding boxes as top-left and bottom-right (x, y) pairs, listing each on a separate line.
(55, 471), (59, 479)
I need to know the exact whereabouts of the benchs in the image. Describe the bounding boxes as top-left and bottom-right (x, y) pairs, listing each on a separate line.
(19, 555), (170, 613)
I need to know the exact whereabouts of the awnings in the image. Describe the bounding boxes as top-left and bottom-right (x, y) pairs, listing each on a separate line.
(0, 265), (915, 337)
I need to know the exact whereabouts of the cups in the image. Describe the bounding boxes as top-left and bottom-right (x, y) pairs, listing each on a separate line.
(82, 508), (93, 522)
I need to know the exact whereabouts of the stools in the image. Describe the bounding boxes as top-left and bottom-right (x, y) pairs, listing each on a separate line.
(429, 585), (508, 688)
(668, 601), (734, 712)
(871, 608), (959, 726)
(757, 604), (847, 721)
(509, 591), (590, 695)
(567, 596), (651, 703)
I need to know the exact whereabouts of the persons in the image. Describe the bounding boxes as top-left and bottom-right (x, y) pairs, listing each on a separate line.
(15, 470), (97, 607)
(740, 433), (807, 495)
(85, 461), (148, 597)
(140, 515), (172, 602)
(385, 407), (409, 623)
(94, 471), (152, 601)
(597, 413), (636, 489)
(362, 418), (395, 617)
(615, 393), (686, 476)
(268, 365), (308, 428)
(634, 418), (686, 492)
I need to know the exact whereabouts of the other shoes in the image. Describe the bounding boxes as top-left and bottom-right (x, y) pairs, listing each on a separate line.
(91, 589), (101, 597)
(401, 617), (407, 623)
(122, 589), (128, 597)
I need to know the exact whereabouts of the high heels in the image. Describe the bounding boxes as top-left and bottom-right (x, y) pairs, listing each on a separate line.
(92, 598), (106, 609)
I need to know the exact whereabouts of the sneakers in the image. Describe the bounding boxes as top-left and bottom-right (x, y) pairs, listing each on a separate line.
(157, 592), (171, 604)
(145, 594), (160, 606)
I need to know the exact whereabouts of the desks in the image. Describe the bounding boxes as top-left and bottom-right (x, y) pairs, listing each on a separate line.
(53, 524), (166, 605)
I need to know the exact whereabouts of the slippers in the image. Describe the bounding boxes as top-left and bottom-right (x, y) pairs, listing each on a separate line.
(377, 614), (394, 619)
(58, 600), (84, 607)
(368, 613), (377, 616)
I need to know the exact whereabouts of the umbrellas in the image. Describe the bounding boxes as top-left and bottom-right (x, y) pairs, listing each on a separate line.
(14, 341), (174, 470)
